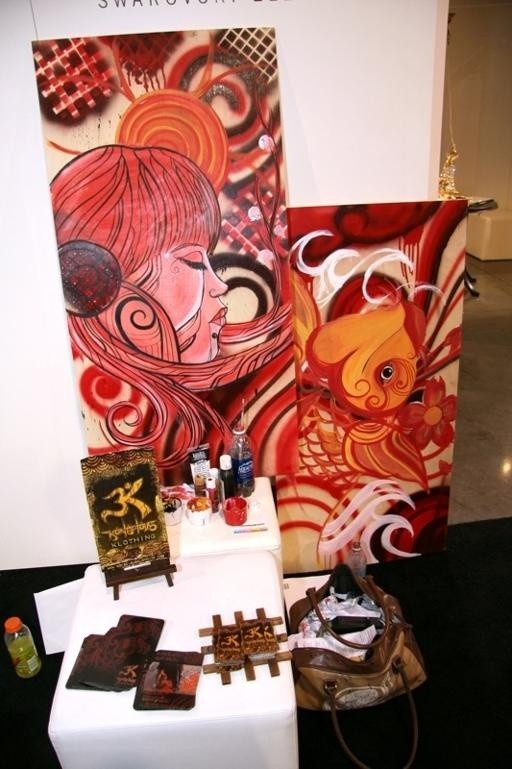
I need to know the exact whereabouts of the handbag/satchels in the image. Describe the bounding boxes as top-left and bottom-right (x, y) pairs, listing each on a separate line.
(289, 562), (428, 712)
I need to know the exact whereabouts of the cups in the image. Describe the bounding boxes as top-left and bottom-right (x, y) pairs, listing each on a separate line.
(223, 497), (248, 526)
(163, 496), (183, 527)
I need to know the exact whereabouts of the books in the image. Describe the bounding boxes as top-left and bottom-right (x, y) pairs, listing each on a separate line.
(212, 626), (246, 666)
(242, 621), (281, 664)
(79, 444), (171, 573)
(63, 614), (205, 712)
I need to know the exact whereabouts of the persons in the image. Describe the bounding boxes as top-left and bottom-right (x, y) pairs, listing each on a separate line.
(50, 143), (293, 471)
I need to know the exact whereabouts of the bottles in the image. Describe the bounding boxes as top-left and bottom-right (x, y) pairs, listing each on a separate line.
(195, 426), (256, 514)
(3, 617), (42, 679)
